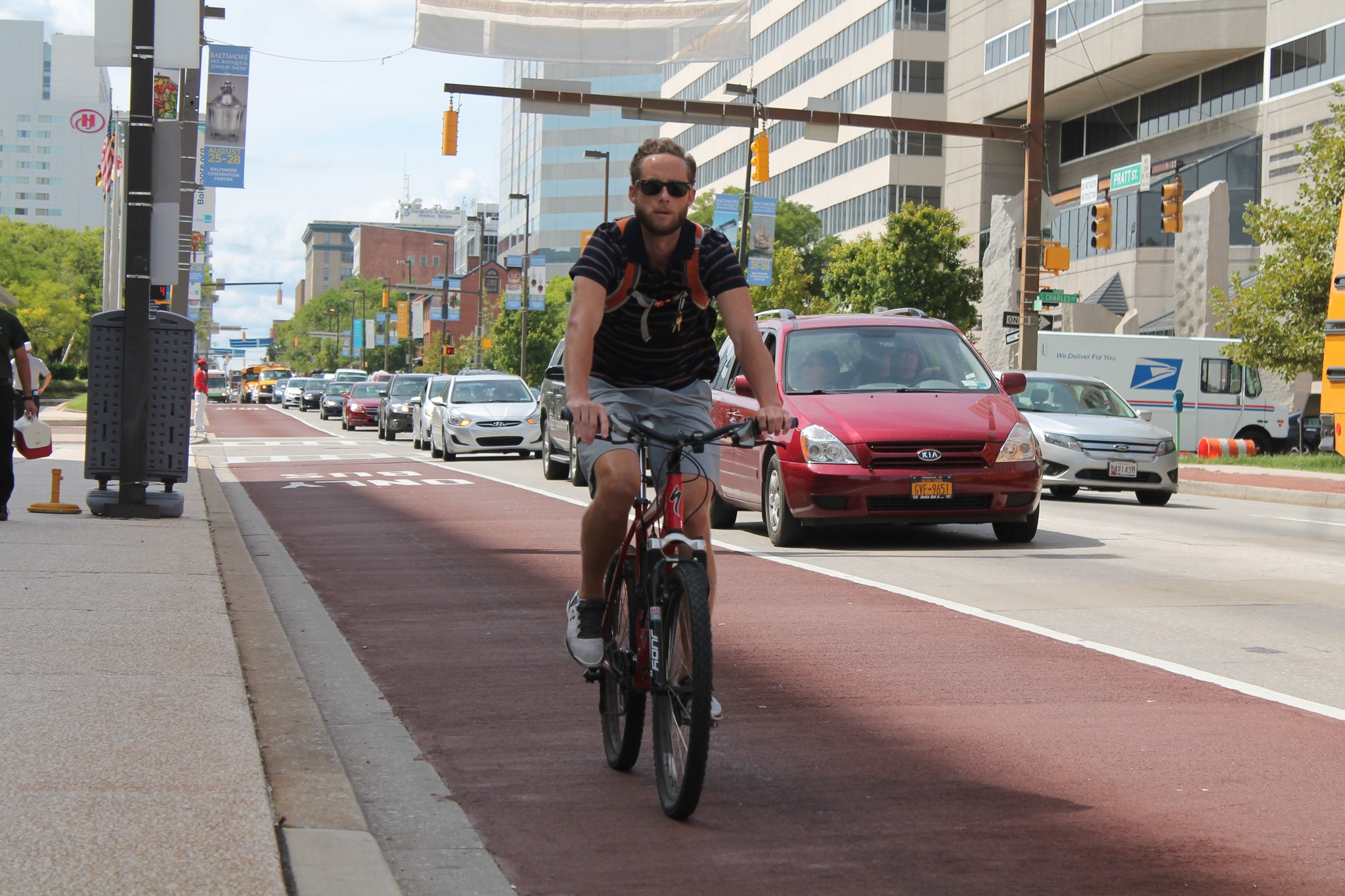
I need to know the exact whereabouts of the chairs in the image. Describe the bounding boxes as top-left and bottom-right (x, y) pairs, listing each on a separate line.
(292, 382), (298, 387)
(357, 388), (367, 396)
(435, 387), (505, 401)
(281, 374), (286, 377)
(801, 351), (841, 391)
(338, 377), (345, 382)
(331, 388), (339, 394)
(1009, 387), (1079, 410)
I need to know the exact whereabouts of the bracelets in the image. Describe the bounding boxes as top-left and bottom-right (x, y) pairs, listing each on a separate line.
(22, 395), (34, 402)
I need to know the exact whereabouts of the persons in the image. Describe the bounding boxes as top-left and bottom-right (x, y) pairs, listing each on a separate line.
(0, 307), (54, 521)
(195, 358), (209, 433)
(794, 336), (951, 396)
(562, 138), (791, 727)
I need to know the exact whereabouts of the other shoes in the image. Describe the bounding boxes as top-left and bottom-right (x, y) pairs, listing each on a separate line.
(0, 503), (10, 521)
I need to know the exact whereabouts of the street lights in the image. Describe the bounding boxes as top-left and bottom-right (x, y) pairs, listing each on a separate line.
(354, 289), (366, 371)
(433, 239), (449, 374)
(466, 216), (486, 370)
(508, 193), (530, 378)
(377, 276), (391, 371)
(396, 259), (413, 374)
(723, 83), (758, 272)
(330, 304), (339, 370)
(583, 150), (609, 222)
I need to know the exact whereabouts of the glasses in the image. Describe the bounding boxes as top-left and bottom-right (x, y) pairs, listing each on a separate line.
(634, 180), (692, 198)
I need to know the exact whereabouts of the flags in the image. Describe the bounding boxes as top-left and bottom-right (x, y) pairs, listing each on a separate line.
(96, 108), (123, 202)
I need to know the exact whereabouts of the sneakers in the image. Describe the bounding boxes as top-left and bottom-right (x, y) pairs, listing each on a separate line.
(195, 427), (206, 431)
(564, 589), (606, 667)
(675, 682), (723, 721)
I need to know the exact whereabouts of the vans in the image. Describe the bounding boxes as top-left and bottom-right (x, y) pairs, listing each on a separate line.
(1036, 329), (1288, 455)
(1317, 188), (1345, 456)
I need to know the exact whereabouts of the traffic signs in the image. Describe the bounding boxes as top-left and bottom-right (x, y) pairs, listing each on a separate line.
(1002, 311), (1054, 331)
(1005, 325), (1040, 346)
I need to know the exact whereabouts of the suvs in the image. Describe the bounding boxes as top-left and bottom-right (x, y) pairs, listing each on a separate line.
(538, 336), (589, 487)
(708, 306), (1045, 547)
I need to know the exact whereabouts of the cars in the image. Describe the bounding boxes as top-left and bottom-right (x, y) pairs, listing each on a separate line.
(1288, 411), (1321, 456)
(430, 375), (542, 462)
(205, 361), (541, 451)
(991, 369), (1179, 507)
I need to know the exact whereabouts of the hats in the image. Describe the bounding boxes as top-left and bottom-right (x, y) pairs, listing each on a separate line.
(24, 342), (31, 350)
(197, 359), (206, 365)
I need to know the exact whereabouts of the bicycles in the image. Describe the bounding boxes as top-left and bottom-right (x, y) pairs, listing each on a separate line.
(558, 406), (800, 821)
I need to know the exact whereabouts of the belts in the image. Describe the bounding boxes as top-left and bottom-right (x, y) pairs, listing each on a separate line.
(196, 390), (204, 393)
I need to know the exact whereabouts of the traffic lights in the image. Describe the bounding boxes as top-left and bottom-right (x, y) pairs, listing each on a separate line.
(152, 285), (172, 305)
(1091, 202), (1113, 250)
(1160, 182), (1183, 234)
(442, 344), (456, 358)
(750, 135), (770, 182)
